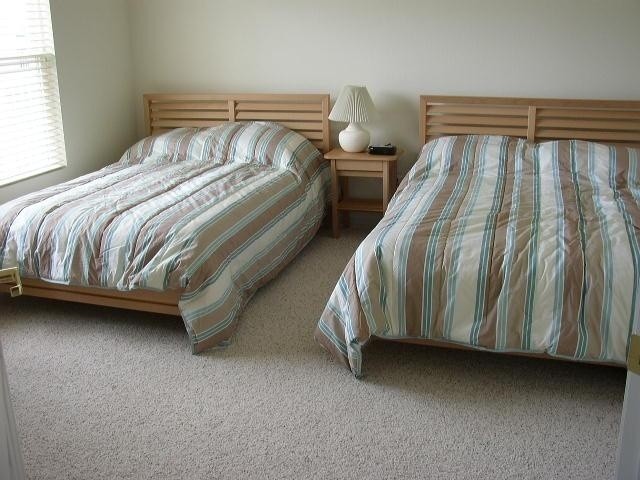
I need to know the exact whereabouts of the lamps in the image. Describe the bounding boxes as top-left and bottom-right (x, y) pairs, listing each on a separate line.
(327, 86), (376, 153)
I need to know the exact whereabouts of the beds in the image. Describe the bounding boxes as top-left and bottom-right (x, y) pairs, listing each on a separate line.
(5, 92), (340, 355)
(315, 95), (639, 379)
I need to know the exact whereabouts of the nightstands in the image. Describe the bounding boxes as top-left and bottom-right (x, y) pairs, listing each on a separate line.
(323, 147), (404, 237)
